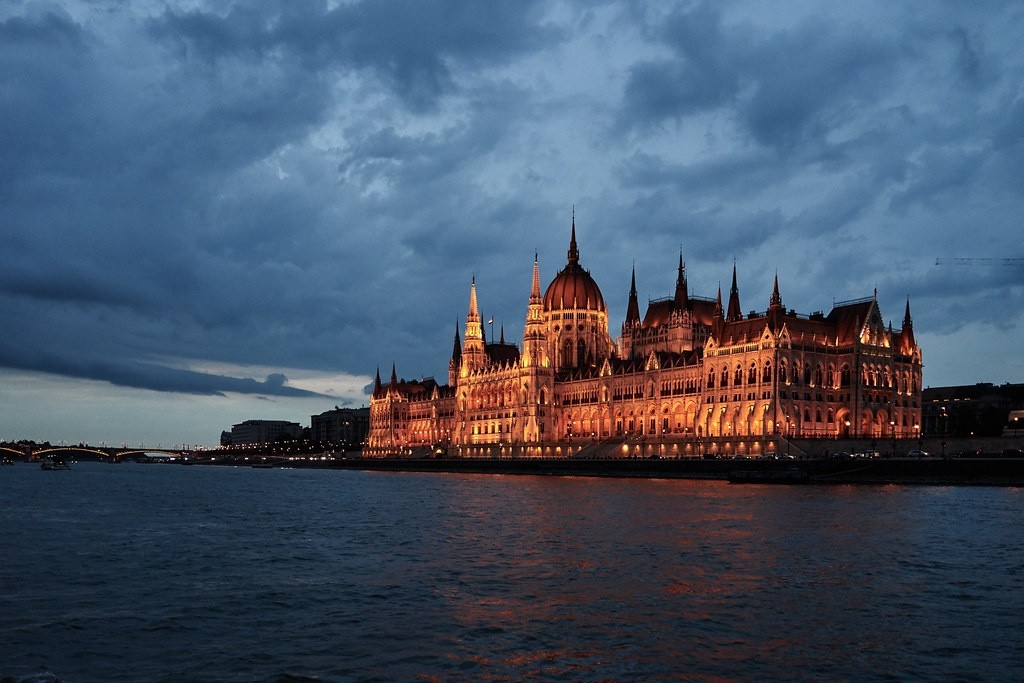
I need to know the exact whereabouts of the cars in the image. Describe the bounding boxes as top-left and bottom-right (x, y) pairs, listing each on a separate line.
(734, 454), (752, 460)
(758, 451), (797, 460)
(832, 448), (880, 460)
(949, 446), (1024, 458)
(647, 454), (666, 460)
(907, 450), (928, 458)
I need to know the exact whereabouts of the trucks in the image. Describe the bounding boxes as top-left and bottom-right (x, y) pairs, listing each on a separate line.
(702, 451), (722, 459)
